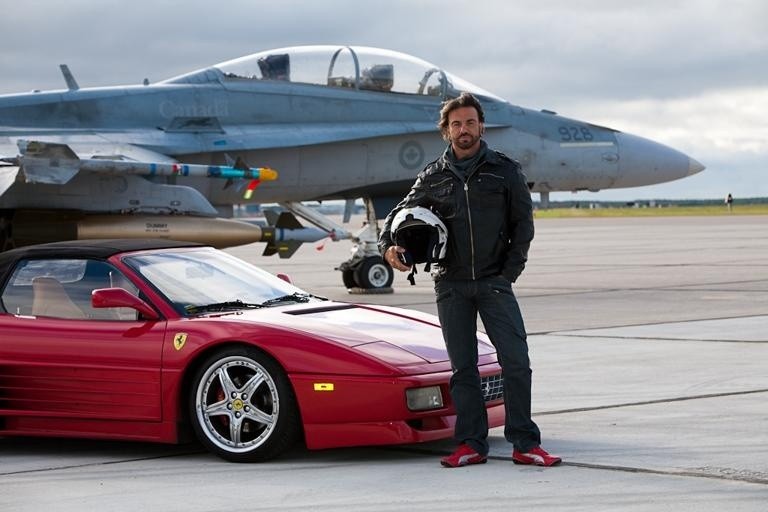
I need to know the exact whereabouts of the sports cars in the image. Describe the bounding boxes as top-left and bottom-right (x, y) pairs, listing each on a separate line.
(2, 237), (507, 463)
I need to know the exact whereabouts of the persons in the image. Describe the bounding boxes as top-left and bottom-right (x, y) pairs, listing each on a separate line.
(377, 93), (561, 467)
(723, 192), (733, 210)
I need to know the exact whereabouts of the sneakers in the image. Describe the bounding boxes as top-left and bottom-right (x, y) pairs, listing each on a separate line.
(440, 443), (488, 469)
(513, 446), (562, 467)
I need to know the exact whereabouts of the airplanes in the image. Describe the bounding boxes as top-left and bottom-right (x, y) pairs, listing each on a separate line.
(0, 43), (707, 294)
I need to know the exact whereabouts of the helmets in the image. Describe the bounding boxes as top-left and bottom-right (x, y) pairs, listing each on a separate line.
(390, 201), (448, 267)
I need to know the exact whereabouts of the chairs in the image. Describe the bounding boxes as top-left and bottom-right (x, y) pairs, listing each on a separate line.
(30, 275), (90, 320)
(108, 271), (140, 320)
